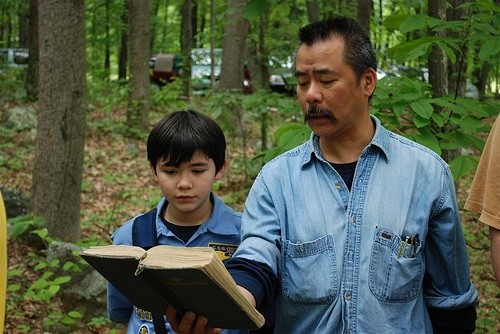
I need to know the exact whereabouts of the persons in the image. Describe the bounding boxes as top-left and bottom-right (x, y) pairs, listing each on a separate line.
(104, 109), (275, 334)
(243, 63), (253, 94)
(165, 15), (480, 334)
(463, 112), (500, 289)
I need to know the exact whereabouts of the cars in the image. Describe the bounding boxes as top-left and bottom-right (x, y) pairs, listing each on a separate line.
(190, 47), (227, 91)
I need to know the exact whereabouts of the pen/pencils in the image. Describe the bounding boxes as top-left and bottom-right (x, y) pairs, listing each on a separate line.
(296, 241), (302, 259)
(399, 234), (420, 258)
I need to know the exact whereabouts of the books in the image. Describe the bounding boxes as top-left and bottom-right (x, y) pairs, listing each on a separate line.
(78, 244), (265, 330)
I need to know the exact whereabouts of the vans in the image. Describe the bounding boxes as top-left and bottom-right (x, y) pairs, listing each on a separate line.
(1, 46), (32, 71)
